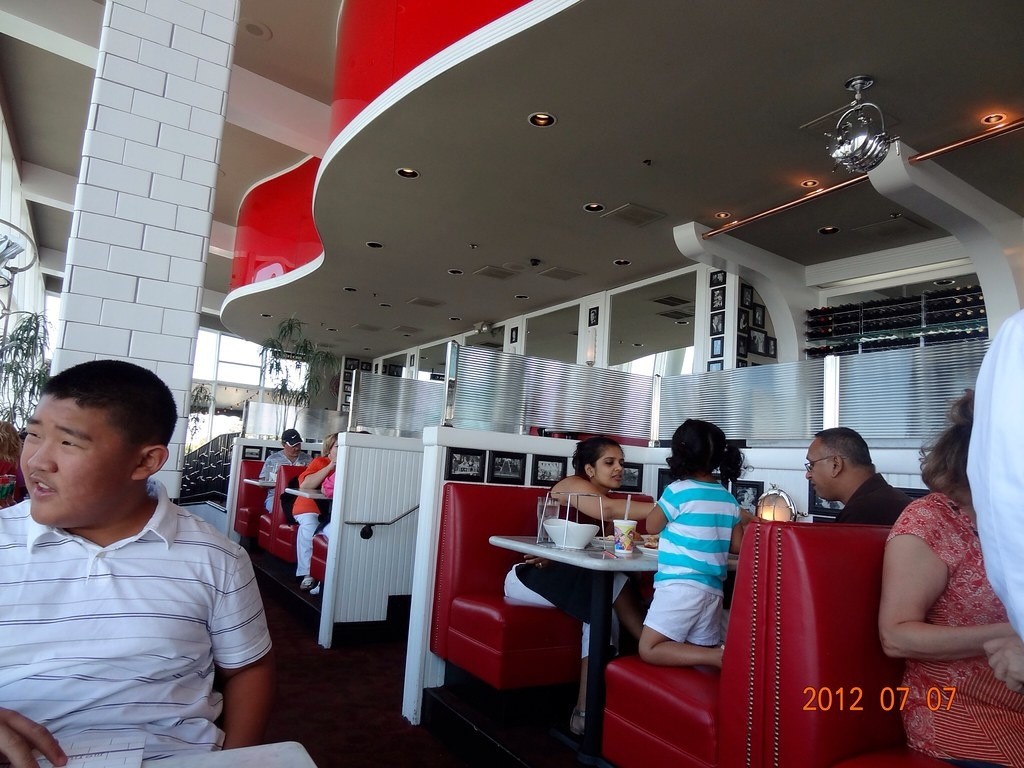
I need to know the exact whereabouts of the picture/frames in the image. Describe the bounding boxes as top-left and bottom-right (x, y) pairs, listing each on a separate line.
(444, 447), (764, 518)
(265, 447), (284, 460)
(708, 270), (777, 372)
(808, 479), (846, 515)
(589, 306), (599, 327)
(510, 327), (518, 343)
(241, 445), (263, 459)
(312, 451), (321, 459)
(341, 355), (414, 413)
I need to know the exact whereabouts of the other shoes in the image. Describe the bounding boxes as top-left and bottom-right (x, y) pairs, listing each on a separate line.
(300, 577), (313, 589)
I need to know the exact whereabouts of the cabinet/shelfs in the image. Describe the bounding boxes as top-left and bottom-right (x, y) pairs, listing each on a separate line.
(805, 289), (988, 360)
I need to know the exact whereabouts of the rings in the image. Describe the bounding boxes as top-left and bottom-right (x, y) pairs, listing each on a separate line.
(539, 563), (543, 568)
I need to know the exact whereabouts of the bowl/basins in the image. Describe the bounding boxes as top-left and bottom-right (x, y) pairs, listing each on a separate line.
(543, 519), (600, 549)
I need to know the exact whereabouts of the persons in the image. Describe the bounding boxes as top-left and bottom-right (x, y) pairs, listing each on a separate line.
(500, 436), (646, 734)
(876, 388), (1024, 768)
(591, 311), (596, 323)
(261, 429), (313, 512)
(0, 359), (272, 768)
(294, 431), (371, 594)
(965, 309), (1024, 692)
(804, 426), (915, 526)
(639, 418), (754, 667)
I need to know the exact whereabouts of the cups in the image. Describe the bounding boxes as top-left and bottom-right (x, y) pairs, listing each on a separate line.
(265, 466), (274, 482)
(613, 520), (637, 554)
(537, 497), (560, 543)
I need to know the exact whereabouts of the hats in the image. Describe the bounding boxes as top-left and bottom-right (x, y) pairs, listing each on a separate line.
(281, 429), (304, 448)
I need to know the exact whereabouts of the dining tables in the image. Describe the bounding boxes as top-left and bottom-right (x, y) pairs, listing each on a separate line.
(490, 536), (738, 758)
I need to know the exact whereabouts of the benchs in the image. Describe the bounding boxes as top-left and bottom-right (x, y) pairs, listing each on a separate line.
(429, 482), (655, 699)
(602, 520), (950, 767)
(234, 461), (329, 602)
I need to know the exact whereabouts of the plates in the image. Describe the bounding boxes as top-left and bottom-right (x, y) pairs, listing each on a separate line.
(592, 535), (658, 556)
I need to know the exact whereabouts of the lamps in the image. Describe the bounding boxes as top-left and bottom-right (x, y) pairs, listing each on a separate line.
(586, 328), (596, 367)
(757, 483), (798, 523)
(822, 74), (900, 174)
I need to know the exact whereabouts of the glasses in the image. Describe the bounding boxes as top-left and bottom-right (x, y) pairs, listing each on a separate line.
(804, 456), (847, 472)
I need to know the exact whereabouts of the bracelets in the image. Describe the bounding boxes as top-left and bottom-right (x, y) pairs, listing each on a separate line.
(654, 501), (658, 508)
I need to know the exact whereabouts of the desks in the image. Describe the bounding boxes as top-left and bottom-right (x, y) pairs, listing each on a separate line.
(142, 741), (317, 768)
(285, 488), (334, 499)
(243, 478), (276, 487)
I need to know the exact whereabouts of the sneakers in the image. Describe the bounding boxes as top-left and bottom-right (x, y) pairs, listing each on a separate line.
(309, 581), (320, 594)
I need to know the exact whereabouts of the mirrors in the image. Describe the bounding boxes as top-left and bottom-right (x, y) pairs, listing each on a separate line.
(381, 267), (697, 384)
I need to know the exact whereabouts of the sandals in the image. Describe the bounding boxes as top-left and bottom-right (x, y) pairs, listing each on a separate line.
(569, 705), (586, 737)
